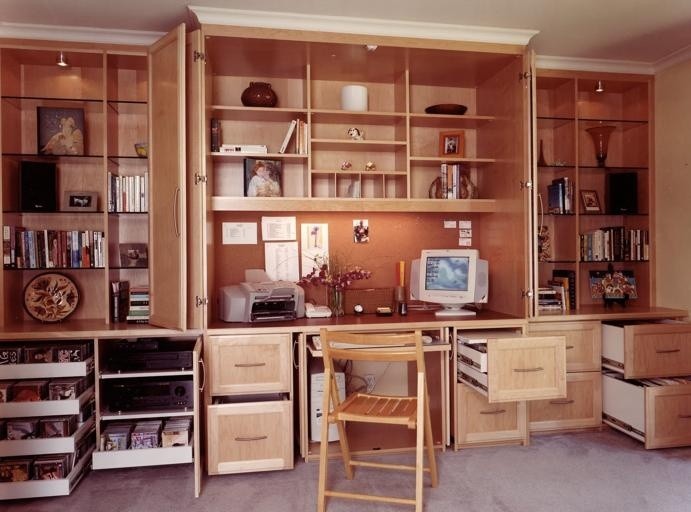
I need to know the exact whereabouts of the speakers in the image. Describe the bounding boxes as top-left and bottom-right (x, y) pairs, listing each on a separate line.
(476, 260), (489, 303)
(607, 171), (639, 216)
(411, 258), (421, 301)
(20, 160), (58, 213)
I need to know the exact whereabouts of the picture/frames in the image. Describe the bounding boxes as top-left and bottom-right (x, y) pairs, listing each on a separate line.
(439, 131), (465, 159)
(243, 156), (284, 198)
(580, 189), (603, 215)
(65, 190), (99, 213)
(35, 105), (86, 157)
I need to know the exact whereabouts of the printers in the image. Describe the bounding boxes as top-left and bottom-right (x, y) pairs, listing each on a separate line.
(219, 269), (305, 323)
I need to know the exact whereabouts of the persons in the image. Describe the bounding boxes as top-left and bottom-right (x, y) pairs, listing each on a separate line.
(263, 163), (281, 197)
(247, 164), (267, 197)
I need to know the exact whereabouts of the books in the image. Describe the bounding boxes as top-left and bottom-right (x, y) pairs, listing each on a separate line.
(0, 341), (94, 365)
(112, 281), (150, 323)
(3, 224), (106, 269)
(581, 226), (649, 262)
(211, 118), (309, 155)
(538, 269), (576, 312)
(104, 417), (192, 451)
(441, 163), (460, 199)
(0, 400), (95, 441)
(0, 435), (96, 483)
(0, 374), (95, 403)
(547, 176), (574, 214)
(108, 171), (149, 213)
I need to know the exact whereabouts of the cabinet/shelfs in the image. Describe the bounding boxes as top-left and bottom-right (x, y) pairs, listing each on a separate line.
(448, 323), (606, 454)
(533, 67), (661, 314)
(185, 21), (534, 329)
(199, 334), (306, 476)
(0, 339), (199, 507)
(603, 322), (691, 450)
(0, 34), (191, 333)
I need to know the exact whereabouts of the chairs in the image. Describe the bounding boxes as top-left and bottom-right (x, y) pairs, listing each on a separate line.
(314, 327), (436, 512)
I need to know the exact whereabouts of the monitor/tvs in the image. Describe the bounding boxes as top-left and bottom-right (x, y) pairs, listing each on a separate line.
(419, 249), (478, 316)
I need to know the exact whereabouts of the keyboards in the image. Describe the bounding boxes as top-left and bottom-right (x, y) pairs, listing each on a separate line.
(312, 333), (435, 351)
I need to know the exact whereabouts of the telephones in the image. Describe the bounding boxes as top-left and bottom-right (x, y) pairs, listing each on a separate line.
(305, 302), (333, 319)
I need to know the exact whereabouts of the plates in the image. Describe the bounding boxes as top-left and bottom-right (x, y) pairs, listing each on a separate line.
(425, 104), (468, 115)
(22, 272), (80, 324)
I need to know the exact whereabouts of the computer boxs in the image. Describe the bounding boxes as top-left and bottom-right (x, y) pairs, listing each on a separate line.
(308, 364), (346, 444)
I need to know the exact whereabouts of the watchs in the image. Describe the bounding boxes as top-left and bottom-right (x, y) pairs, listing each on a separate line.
(354, 305), (363, 316)
